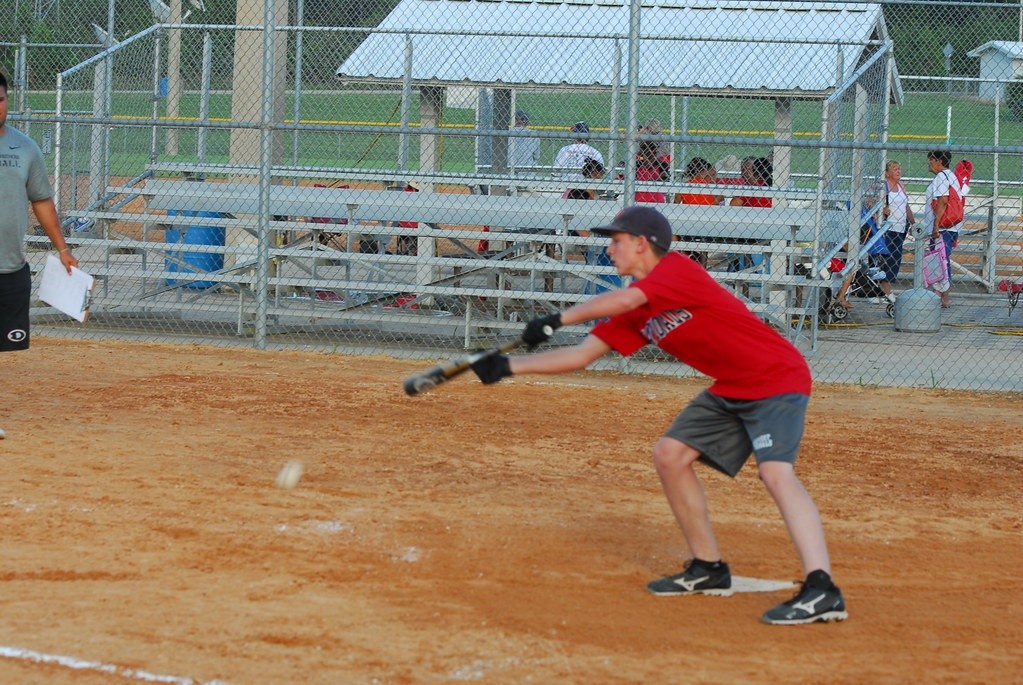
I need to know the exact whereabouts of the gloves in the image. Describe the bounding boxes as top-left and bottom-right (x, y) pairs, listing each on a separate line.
(468, 348), (514, 385)
(521, 313), (563, 351)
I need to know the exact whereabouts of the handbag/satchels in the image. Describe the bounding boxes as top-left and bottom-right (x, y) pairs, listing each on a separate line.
(931, 171), (963, 229)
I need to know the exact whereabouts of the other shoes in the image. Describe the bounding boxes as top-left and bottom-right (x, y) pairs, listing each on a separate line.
(881, 293), (896, 304)
(868, 296), (879, 304)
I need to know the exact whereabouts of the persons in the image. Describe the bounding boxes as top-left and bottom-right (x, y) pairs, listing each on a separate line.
(508, 109), (540, 256)
(617, 118), (669, 203)
(672, 157), (725, 243)
(540, 122), (605, 255)
(729, 151), (772, 246)
(0, 72), (79, 440)
(468, 206), (849, 626)
(876, 160), (915, 295)
(925, 151), (961, 308)
(562, 156), (605, 264)
(827, 257), (854, 309)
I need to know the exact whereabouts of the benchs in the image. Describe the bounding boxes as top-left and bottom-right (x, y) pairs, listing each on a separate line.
(28, 162), (1022, 356)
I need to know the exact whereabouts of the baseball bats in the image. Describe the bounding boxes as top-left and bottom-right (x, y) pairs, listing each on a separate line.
(402, 325), (552, 395)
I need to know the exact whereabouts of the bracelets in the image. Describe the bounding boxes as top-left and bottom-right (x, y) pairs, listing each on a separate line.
(60, 248), (68, 253)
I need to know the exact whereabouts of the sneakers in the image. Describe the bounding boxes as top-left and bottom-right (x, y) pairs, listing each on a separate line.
(647, 563), (732, 596)
(761, 581), (847, 624)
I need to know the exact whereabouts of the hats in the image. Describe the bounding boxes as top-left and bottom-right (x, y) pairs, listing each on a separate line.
(569, 121), (589, 142)
(715, 155), (741, 173)
(591, 206), (672, 252)
(515, 111), (528, 123)
